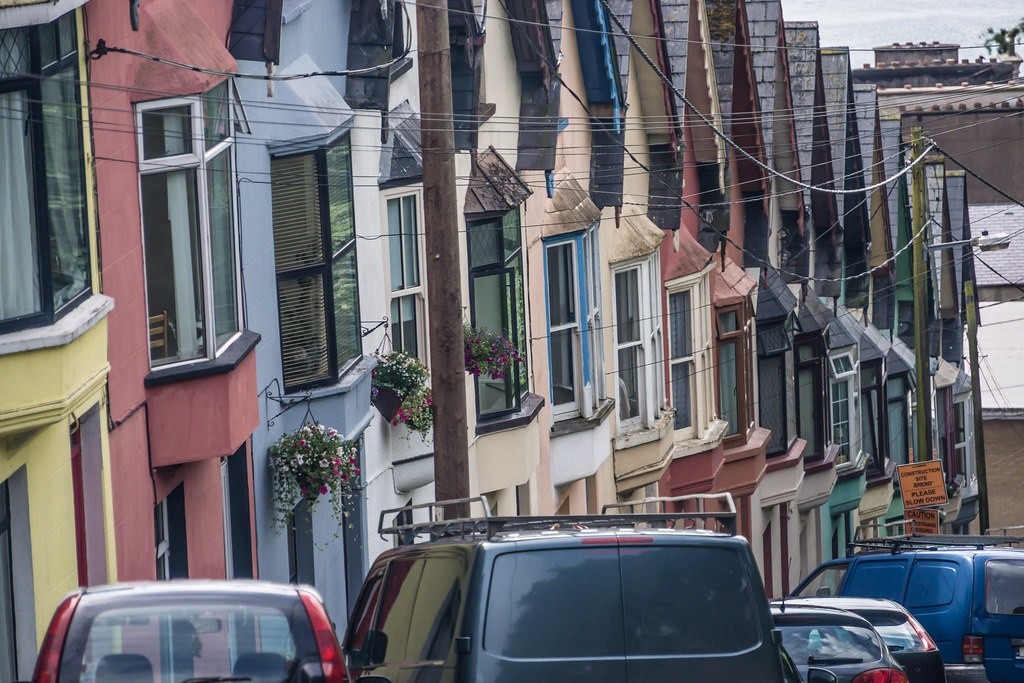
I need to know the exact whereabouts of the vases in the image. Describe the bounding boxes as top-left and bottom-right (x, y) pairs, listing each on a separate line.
(290, 467), (323, 502)
(371, 387), (411, 424)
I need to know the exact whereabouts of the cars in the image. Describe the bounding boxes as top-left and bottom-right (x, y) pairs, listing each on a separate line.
(769, 608), (913, 683)
(33, 577), (394, 682)
(770, 595), (948, 683)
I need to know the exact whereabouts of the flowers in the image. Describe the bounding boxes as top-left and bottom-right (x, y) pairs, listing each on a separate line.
(462, 322), (521, 383)
(370, 349), (435, 437)
(267, 422), (363, 542)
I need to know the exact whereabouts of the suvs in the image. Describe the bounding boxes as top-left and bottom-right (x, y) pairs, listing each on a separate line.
(792, 518), (1023, 683)
(341, 494), (793, 682)
